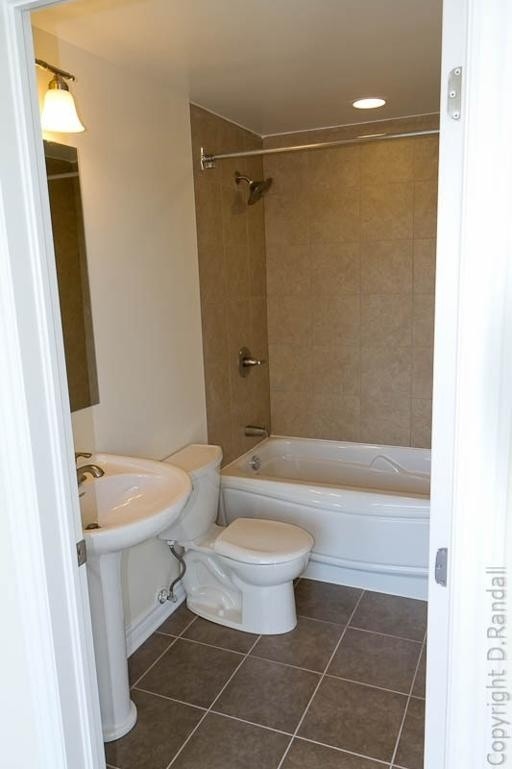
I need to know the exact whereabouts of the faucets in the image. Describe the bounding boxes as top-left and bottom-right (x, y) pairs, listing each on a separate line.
(74, 452), (106, 482)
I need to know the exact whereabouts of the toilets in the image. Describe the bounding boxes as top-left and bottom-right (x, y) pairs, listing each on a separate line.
(160, 443), (316, 637)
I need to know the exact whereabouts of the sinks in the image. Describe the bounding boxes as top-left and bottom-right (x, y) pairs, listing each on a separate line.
(78, 452), (193, 557)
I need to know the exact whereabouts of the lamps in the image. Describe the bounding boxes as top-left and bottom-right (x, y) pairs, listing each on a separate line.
(31, 53), (89, 140)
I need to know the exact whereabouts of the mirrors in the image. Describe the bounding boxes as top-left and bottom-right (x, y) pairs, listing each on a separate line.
(40, 140), (104, 415)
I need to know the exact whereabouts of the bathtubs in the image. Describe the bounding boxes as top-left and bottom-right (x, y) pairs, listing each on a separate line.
(218, 433), (433, 603)
